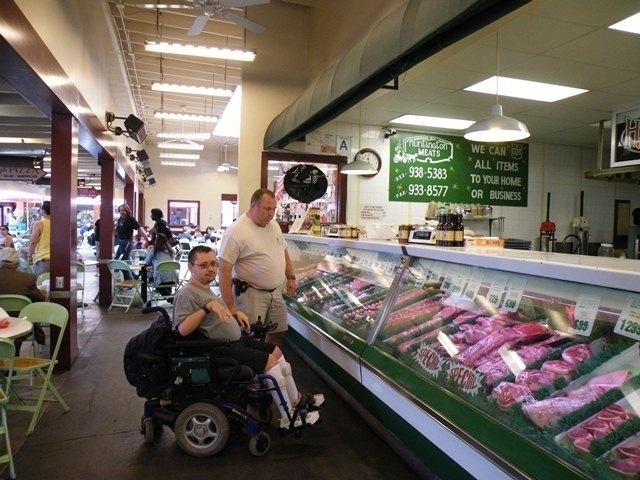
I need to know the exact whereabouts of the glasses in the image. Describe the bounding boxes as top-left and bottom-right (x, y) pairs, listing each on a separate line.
(192, 260), (217, 270)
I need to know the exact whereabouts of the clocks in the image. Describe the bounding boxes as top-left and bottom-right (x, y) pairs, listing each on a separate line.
(355, 148), (382, 177)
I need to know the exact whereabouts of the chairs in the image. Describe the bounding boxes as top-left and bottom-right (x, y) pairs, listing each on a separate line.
(7, 302), (72, 435)
(0, 389), (17, 480)
(71, 226), (207, 314)
(0, 294), (40, 386)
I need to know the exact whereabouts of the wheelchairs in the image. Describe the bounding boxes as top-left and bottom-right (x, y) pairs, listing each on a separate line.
(133, 300), (323, 458)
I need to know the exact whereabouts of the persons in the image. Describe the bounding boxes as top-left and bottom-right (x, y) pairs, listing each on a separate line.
(1, 318), (10, 328)
(80, 222), (95, 246)
(0, 247), (50, 378)
(77, 222), (82, 228)
(93, 219), (101, 276)
(172, 245), (325, 431)
(14, 218), (27, 231)
(219, 189), (296, 347)
(1, 225), (14, 249)
(29, 201), (51, 277)
(115, 204), (225, 303)
(17, 258), (32, 272)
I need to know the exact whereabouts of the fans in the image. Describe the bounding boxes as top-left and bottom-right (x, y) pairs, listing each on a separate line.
(187, 0), (270, 36)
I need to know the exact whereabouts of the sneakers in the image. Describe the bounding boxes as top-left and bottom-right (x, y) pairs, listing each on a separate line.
(279, 407), (321, 429)
(292, 392), (326, 410)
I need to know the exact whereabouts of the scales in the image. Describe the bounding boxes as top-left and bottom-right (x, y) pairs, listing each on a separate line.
(327, 224), (360, 239)
(408, 227), (437, 245)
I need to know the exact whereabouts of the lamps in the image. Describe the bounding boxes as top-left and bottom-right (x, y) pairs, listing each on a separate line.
(144, 39), (256, 166)
(341, 105), (378, 176)
(464, 31), (530, 144)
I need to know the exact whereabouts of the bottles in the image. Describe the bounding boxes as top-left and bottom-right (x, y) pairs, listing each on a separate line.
(311, 225), (358, 238)
(437, 212), (464, 247)
(427, 202), (493, 217)
(134, 252), (140, 265)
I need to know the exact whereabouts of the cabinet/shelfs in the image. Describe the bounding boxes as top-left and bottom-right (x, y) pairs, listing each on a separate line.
(280, 233), (640, 480)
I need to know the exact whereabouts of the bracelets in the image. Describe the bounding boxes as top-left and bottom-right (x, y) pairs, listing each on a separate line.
(286, 274), (296, 279)
(202, 304), (211, 315)
